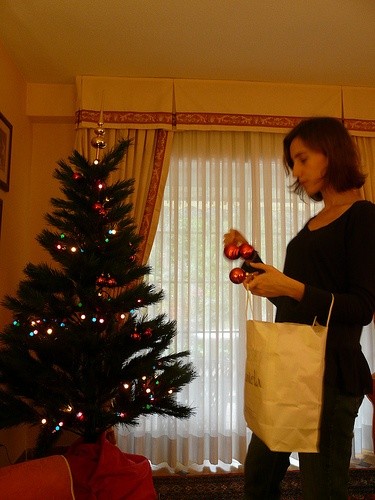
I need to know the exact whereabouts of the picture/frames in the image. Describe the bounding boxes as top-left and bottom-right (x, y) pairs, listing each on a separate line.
(0, 111), (13, 192)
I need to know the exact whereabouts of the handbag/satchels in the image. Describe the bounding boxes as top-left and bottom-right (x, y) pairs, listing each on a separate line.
(241, 276), (336, 456)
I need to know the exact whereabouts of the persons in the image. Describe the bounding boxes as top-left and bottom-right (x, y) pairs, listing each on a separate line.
(223, 116), (375, 500)
